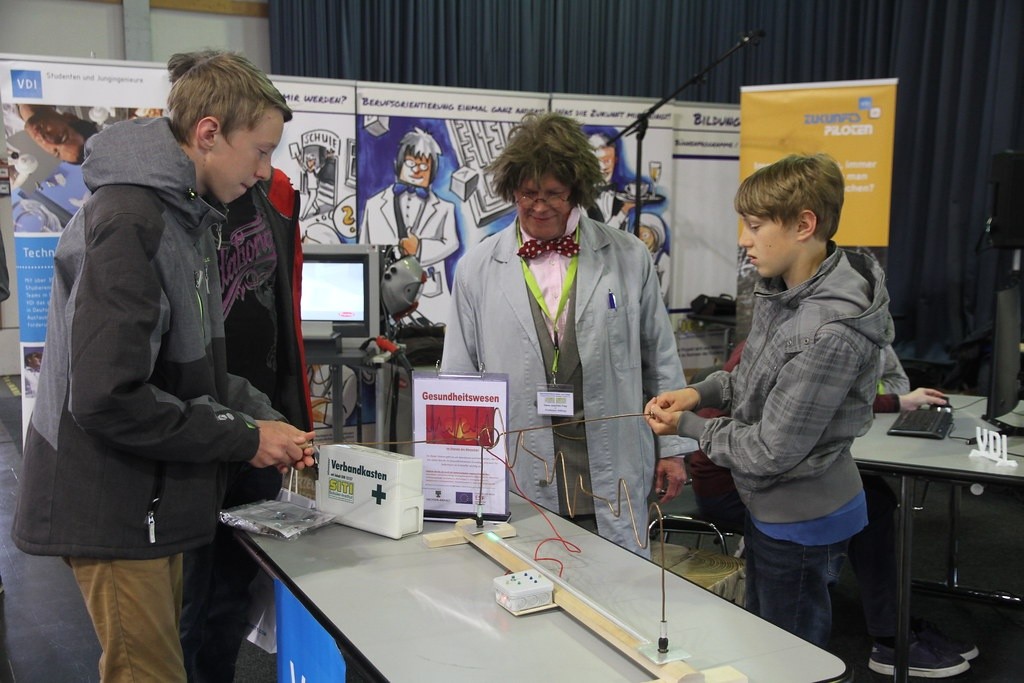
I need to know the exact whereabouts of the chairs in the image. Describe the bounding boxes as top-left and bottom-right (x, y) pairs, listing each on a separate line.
(648, 364), (734, 557)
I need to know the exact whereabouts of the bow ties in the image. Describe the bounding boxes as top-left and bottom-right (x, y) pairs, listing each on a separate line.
(516, 236), (580, 259)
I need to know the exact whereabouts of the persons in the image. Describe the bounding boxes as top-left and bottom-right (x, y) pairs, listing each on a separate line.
(25, 352), (42, 398)
(645, 153), (978, 682)
(11, 53), (317, 683)
(2, 104), (99, 165)
(442, 114), (699, 559)
(0, 230), (11, 595)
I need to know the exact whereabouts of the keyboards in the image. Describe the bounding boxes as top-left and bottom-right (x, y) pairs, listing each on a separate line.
(885, 408), (954, 440)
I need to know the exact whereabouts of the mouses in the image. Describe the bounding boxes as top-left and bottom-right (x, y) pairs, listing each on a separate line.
(917, 396), (949, 412)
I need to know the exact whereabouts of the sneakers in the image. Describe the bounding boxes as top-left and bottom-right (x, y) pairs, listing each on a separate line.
(914, 617), (980, 660)
(867, 636), (971, 678)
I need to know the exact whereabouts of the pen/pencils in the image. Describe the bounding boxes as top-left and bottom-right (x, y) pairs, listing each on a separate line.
(607, 288), (618, 311)
(660, 477), (693, 496)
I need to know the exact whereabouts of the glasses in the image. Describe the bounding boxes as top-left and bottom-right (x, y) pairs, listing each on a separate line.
(514, 193), (566, 210)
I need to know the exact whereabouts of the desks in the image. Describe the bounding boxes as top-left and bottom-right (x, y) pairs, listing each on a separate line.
(305, 346), (376, 443)
(850, 394), (1024, 683)
(231, 492), (849, 683)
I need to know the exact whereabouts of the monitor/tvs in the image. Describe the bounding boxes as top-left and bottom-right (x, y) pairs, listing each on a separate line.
(986, 283), (1024, 427)
(295, 243), (384, 341)
